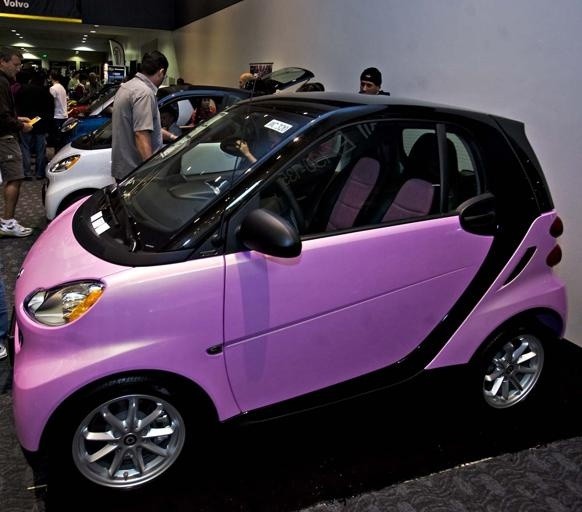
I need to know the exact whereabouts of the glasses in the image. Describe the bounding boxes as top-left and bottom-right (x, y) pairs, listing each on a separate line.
(10, 60), (23, 69)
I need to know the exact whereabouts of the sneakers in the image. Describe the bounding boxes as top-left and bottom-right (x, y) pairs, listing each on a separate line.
(0, 217), (34, 237)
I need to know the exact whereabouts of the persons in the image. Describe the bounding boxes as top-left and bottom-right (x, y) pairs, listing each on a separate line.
(13, 61), (102, 175)
(234, 126), (313, 216)
(160, 106), (183, 137)
(113, 46), (179, 188)
(238, 72), (254, 89)
(358, 67), (391, 99)
(0, 45), (34, 238)
(191, 101), (216, 128)
(1, 274), (10, 359)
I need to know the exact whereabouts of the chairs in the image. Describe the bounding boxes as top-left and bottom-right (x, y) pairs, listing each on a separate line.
(310, 122), (394, 236)
(376, 131), (457, 227)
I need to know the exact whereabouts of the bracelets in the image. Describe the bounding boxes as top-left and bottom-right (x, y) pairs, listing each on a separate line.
(170, 134), (174, 140)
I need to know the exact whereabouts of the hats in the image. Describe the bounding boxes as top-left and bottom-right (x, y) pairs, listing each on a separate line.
(360, 67), (382, 86)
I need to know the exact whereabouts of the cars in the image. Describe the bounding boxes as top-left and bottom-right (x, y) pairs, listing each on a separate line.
(60, 79), (195, 144)
(6, 90), (572, 505)
(39, 64), (319, 225)
